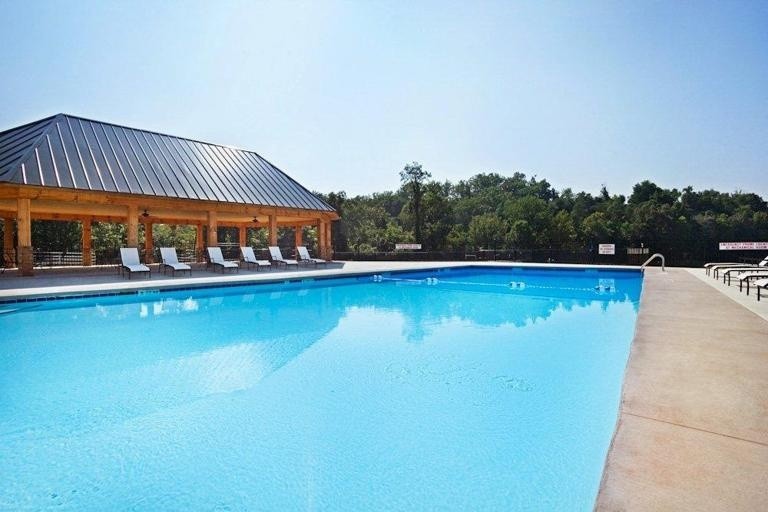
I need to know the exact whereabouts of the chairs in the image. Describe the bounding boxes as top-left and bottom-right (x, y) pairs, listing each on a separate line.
(117, 246), (327, 281)
(703, 255), (768, 301)
